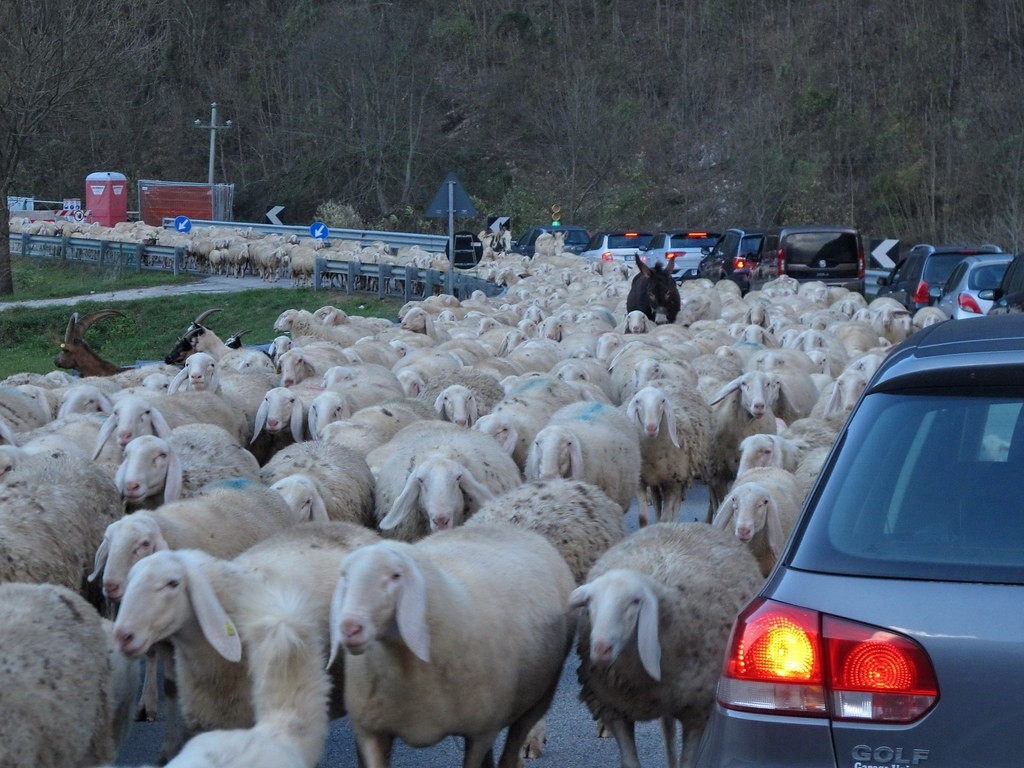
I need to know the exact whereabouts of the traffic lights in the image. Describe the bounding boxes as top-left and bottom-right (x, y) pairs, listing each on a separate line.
(551, 204), (561, 227)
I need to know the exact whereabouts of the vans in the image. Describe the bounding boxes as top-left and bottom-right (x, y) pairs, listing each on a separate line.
(746, 225), (865, 298)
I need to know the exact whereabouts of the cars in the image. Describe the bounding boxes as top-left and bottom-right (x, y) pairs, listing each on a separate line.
(511, 225), (592, 259)
(745, 229), (780, 292)
(576, 231), (653, 265)
(977, 251), (1024, 316)
(928, 253), (1014, 320)
(688, 312), (1024, 768)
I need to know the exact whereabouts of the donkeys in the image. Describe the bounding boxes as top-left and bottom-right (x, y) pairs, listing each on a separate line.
(626, 252), (681, 326)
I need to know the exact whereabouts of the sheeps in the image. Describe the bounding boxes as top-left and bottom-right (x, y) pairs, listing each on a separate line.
(0, 210), (946, 767)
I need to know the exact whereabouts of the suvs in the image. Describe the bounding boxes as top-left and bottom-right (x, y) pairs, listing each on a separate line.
(697, 228), (765, 292)
(639, 228), (722, 281)
(875, 244), (1003, 318)
